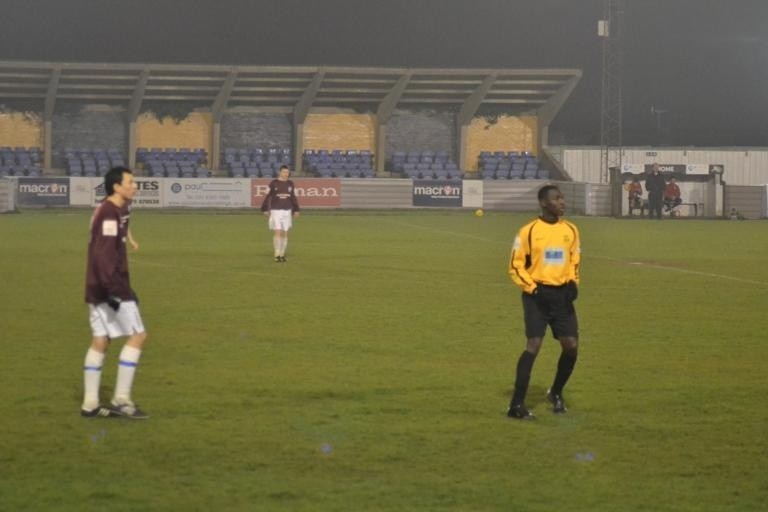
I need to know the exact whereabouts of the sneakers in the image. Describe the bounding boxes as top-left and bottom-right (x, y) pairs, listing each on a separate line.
(508, 400), (537, 421)
(80, 395), (151, 419)
(274, 254), (286, 262)
(546, 385), (568, 414)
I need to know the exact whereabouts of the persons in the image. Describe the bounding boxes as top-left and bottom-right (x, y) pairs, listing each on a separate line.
(507, 184), (582, 420)
(645, 162), (666, 220)
(628, 176), (645, 218)
(664, 176), (683, 219)
(127, 230), (139, 250)
(78, 165), (152, 420)
(260, 164), (300, 263)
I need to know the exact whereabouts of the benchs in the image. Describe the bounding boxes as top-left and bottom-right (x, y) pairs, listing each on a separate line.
(626, 200), (699, 217)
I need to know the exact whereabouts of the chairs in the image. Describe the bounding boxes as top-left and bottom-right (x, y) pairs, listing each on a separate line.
(0, 147), (552, 180)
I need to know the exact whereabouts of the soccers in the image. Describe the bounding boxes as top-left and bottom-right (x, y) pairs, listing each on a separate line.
(475, 209), (484, 218)
